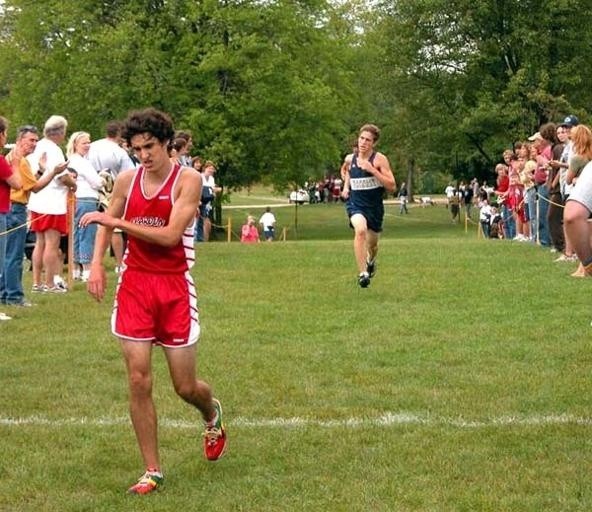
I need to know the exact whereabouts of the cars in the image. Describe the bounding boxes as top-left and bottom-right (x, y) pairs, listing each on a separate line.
(290, 183), (321, 203)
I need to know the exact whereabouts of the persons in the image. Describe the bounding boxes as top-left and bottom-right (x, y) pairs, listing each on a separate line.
(170, 130), (221, 241)
(341, 123), (397, 288)
(79, 107), (226, 494)
(0, 114), (135, 310)
(444, 115), (592, 278)
(398, 186), (409, 214)
(241, 215), (260, 241)
(307, 176), (350, 203)
(260, 207), (276, 241)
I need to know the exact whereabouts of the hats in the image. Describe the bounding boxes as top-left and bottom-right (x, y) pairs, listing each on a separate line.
(560, 114), (579, 127)
(527, 132), (546, 142)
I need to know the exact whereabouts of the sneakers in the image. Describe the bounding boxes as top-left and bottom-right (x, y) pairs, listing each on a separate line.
(0, 298), (38, 320)
(553, 252), (579, 264)
(512, 232), (535, 245)
(31, 275), (67, 296)
(570, 262), (589, 280)
(367, 258), (376, 278)
(71, 270), (94, 282)
(356, 272), (371, 289)
(124, 468), (164, 497)
(202, 397), (228, 462)
